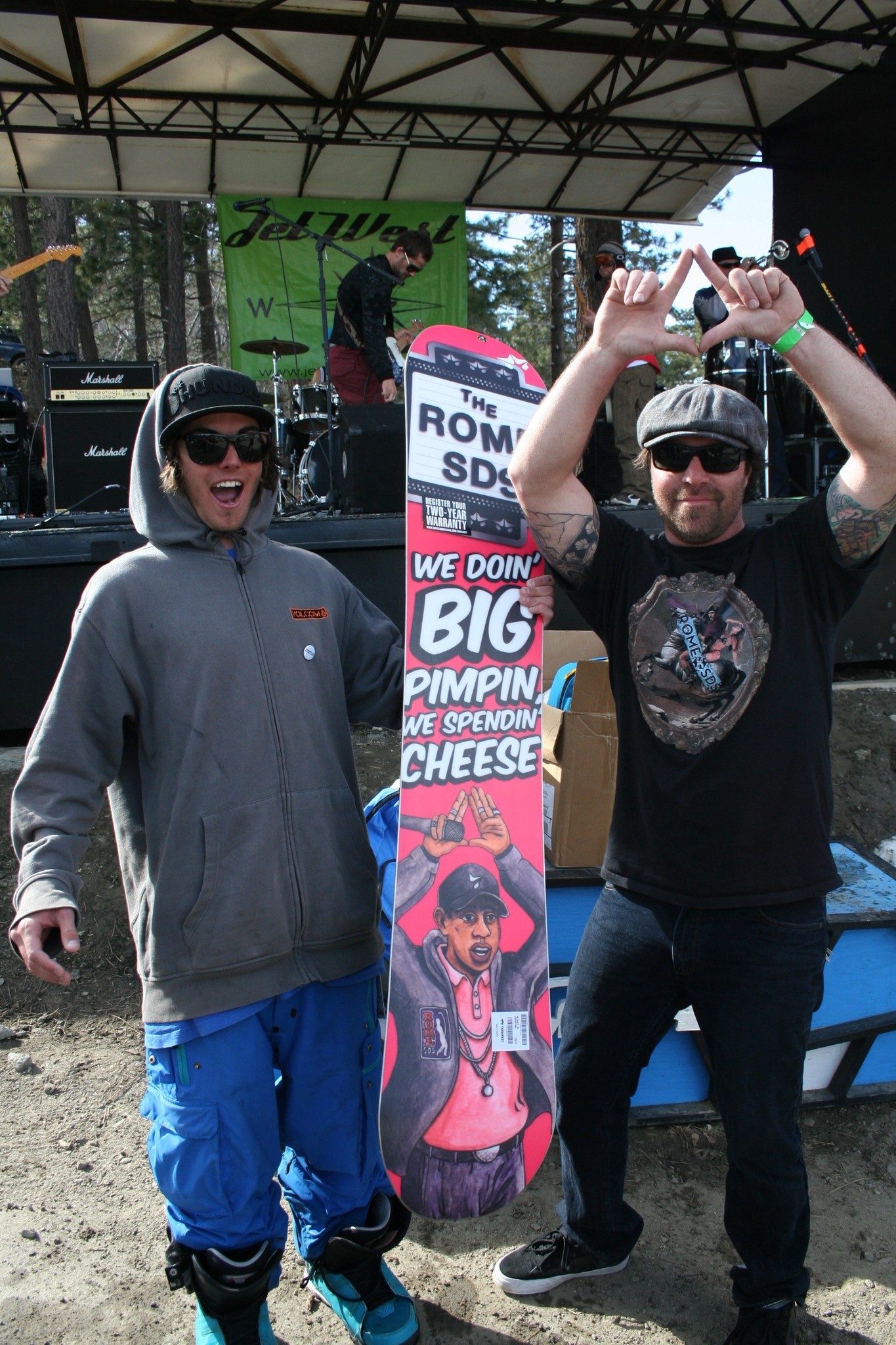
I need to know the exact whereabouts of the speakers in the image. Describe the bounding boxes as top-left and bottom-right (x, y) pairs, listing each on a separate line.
(42, 405), (151, 514)
(575, 423), (623, 500)
(336, 405), (407, 512)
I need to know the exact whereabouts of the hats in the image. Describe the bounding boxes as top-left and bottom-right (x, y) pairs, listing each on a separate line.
(596, 240), (626, 265)
(636, 378), (768, 463)
(157, 365), (277, 452)
(711, 247), (743, 263)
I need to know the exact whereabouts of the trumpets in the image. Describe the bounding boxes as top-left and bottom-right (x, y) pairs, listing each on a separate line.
(751, 236), (791, 271)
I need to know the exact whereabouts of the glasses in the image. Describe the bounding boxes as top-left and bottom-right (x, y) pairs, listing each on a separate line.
(718, 262), (740, 269)
(594, 254), (612, 266)
(402, 251), (424, 273)
(177, 431), (271, 465)
(647, 445), (747, 472)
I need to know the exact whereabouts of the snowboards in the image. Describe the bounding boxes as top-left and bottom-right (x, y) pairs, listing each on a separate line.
(382, 322), (559, 1224)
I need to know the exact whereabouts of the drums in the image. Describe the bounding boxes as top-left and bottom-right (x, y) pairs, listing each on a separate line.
(269, 418), (298, 478)
(297, 425), (342, 506)
(290, 382), (340, 436)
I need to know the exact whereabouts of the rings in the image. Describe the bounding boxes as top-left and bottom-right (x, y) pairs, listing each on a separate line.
(393, 397), (395, 400)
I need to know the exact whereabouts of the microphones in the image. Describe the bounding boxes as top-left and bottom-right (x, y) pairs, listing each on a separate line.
(234, 198), (271, 210)
(799, 229), (824, 271)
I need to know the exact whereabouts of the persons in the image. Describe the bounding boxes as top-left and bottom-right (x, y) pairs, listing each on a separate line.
(580, 243), (662, 507)
(1, 361), (556, 1344)
(326, 231), (432, 403)
(0, 275), (13, 298)
(310, 326), (336, 383)
(693, 246), (761, 386)
(508, 243), (896, 1345)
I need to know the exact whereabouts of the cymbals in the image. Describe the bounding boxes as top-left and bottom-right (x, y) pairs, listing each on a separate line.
(239, 339), (309, 356)
(256, 390), (283, 406)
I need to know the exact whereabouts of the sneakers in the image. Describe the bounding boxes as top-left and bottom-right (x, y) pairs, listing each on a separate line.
(492, 1227), (630, 1294)
(723, 1296), (802, 1345)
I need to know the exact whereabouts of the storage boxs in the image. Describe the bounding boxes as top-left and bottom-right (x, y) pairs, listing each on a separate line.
(525, 627), (623, 870)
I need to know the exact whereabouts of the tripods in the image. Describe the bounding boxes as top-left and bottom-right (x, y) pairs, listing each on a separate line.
(267, 409), (302, 516)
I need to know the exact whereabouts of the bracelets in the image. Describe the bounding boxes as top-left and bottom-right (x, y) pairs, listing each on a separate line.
(767, 307), (814, 355)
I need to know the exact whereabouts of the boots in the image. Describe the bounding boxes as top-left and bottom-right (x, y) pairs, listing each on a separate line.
(305, 1196), (422, 1345)
(164, 1224), (281, 1345)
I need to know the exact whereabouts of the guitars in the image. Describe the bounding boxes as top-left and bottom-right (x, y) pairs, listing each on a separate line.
(1, 242), (83, 284)
(571, 274), (594, 341)
(386, 317), (426, 388)
